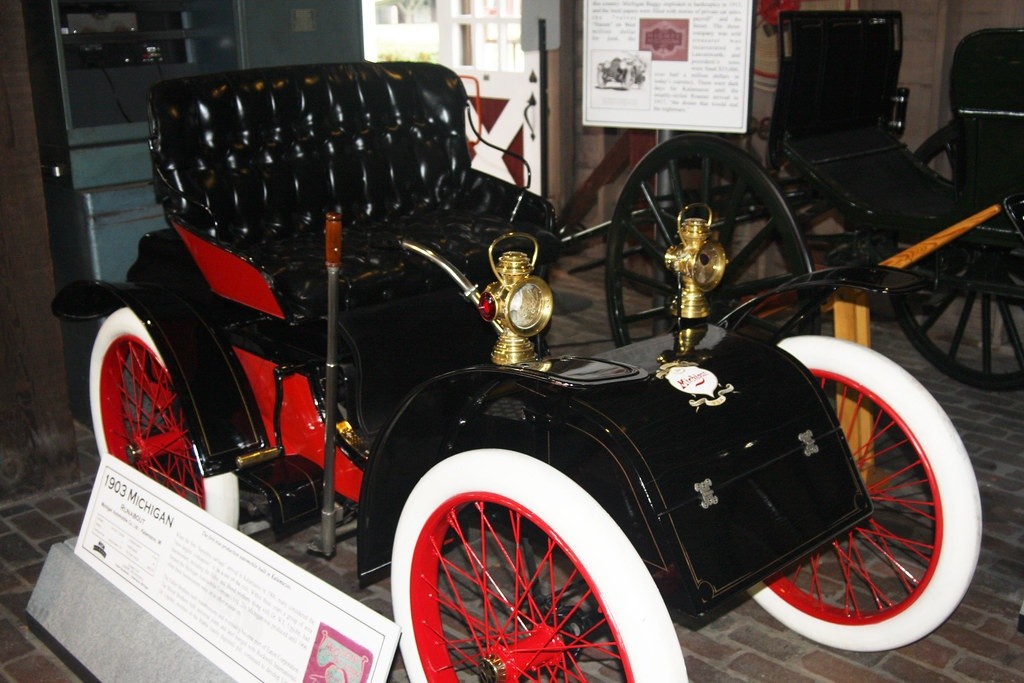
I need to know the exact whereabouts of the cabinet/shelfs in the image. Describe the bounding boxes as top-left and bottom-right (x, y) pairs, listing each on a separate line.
(22, 0), (245, 427)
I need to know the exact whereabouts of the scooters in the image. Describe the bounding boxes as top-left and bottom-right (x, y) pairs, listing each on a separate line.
(88, 60), (983, 681)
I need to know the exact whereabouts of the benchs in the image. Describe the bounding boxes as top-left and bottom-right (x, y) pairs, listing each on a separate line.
(146, 62), (561, 331)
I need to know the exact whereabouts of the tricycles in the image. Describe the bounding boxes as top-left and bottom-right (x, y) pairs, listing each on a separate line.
(603, 7), (1024, 393)
